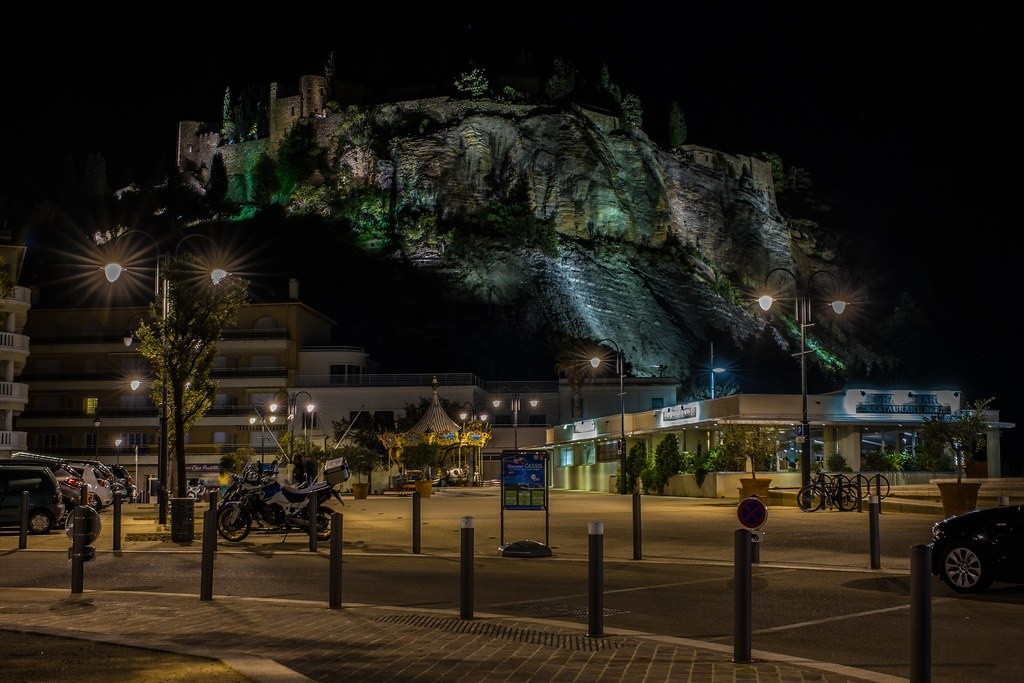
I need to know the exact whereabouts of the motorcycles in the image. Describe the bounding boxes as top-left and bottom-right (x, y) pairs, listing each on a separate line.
(216, 456), (352, 545)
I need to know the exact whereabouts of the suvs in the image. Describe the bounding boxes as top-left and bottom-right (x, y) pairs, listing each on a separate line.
(0, 453), (139, 535)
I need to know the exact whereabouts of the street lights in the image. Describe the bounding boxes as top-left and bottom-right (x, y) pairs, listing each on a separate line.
(270, 391), (316, 465)
(101, 230), (229, 525)
(589, 338), (627, 494)
(492, 386), (541, 451)
(458, 402), (487, 487)
(250, 406), (279, 465)
(756, 267), (852, 509)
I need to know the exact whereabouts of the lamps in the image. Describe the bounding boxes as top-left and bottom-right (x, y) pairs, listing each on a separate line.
(953, 392), (962, 397)
(668, 407), (678, 411)
(653, 410), (664, 416)
(562, 423), (574, 429)
(907, 391), (914, 398)
(858, 390), (866, 397)
(681, 404), (692, 411)
(580, 419), (594, 425)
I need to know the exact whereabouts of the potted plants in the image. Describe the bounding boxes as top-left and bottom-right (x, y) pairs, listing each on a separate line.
(727, 419), (780, 506)
(917, 397), (1002, 517)
(334, 445), (380, 501)
(400, 442), (438, 498)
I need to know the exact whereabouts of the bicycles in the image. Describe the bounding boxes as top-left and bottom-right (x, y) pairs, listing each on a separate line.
(795, 471), (859, 513)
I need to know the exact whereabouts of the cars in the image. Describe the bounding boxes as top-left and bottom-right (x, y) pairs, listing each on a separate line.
(926, 504), (1024, 594)
(402, 470), (422, 490)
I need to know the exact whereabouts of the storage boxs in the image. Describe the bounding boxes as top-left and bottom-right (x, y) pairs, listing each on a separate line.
(324, 457), (350, 484)
